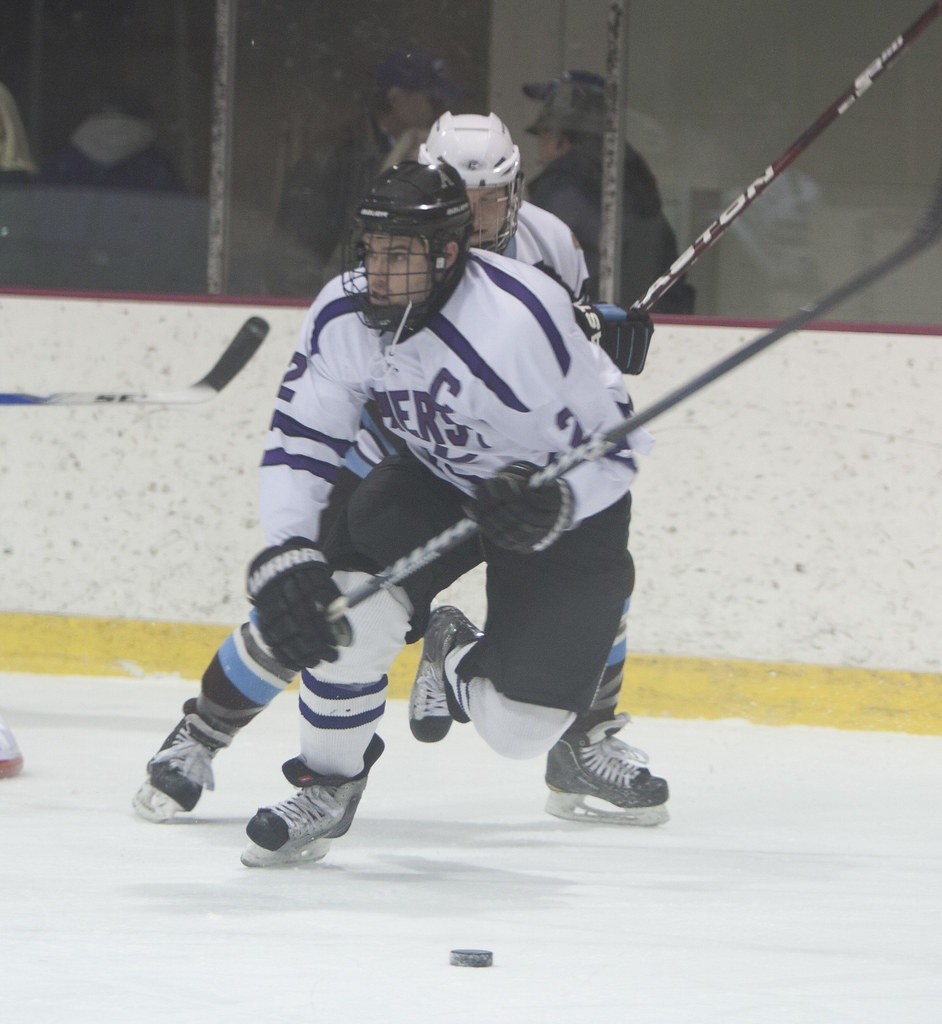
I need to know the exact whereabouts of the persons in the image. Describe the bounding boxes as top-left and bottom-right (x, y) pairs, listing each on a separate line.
(264, 51), (439, 294)
(523, 68), (697, 313)
(1, 75), (198, 293)
(132, 109), (668, 822)
(245, 161), (639, 866)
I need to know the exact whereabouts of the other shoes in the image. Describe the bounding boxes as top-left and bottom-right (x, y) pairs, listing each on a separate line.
(0, 725), (22, 779)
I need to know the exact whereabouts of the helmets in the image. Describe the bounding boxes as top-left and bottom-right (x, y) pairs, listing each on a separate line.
(417, 110), (521, 253)
(339, 157), (474, 334)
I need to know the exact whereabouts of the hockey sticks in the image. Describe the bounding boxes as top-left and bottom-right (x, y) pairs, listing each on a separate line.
(624, 25), (926, 316)
(1, 313), (271, 406)
(320, 204), (940, 625)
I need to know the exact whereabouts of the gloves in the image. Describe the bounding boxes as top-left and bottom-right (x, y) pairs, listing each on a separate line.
(591, 301), (655, 375)
(245, 535), (352, 670)
(460, 460), (576, 555)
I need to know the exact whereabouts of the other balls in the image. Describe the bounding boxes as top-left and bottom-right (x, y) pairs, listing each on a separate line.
(446, 948), (496, 969)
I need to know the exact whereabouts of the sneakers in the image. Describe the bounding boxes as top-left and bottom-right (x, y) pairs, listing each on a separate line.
(408, 607), (484, 743)
(241, 733), (384, 867)
(132, 697), (233, 822)
(544, 712), (670, 828)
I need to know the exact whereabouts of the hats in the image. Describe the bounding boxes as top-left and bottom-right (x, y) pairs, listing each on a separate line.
(522, 70), (605, 101)
(524, 83), (618, 135)
(370, 49), (441, 91)
